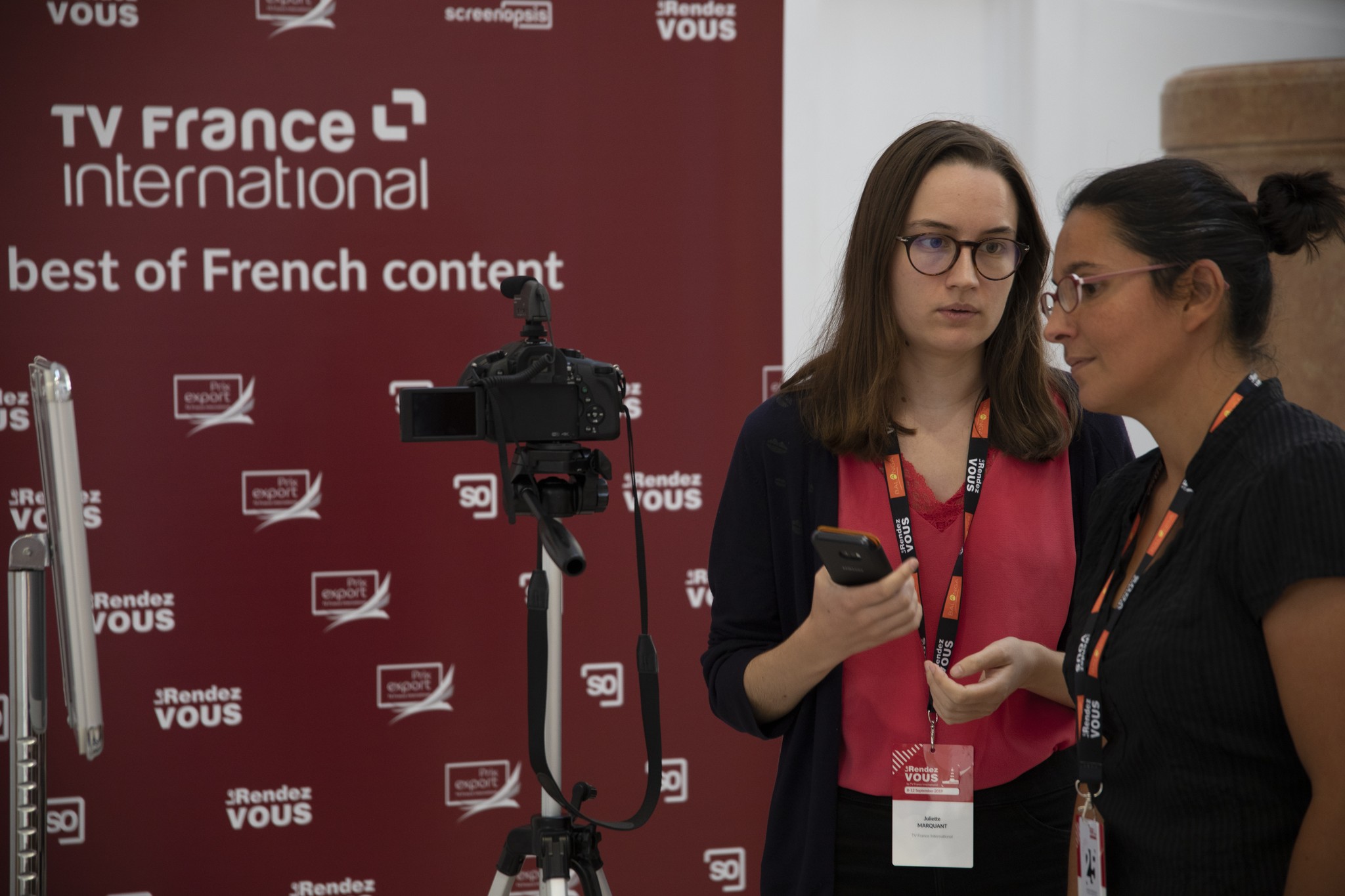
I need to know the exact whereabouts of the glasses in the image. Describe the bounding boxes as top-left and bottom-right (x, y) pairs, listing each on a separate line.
(896, 231), (1030, 281)
(1039, 262), (1229, 322)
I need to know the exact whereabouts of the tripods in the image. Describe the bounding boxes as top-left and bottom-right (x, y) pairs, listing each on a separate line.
(481, 438), (613, 895)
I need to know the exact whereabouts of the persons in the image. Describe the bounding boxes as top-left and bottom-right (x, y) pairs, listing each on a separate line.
(1039, 158), (1345, 895)
(699, 117), (1136, 896)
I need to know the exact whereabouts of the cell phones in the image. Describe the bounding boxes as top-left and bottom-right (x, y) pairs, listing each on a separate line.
(811, 523), (892, 589)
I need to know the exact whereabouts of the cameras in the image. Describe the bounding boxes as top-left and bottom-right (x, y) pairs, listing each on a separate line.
(399, 277), (631, 447)
